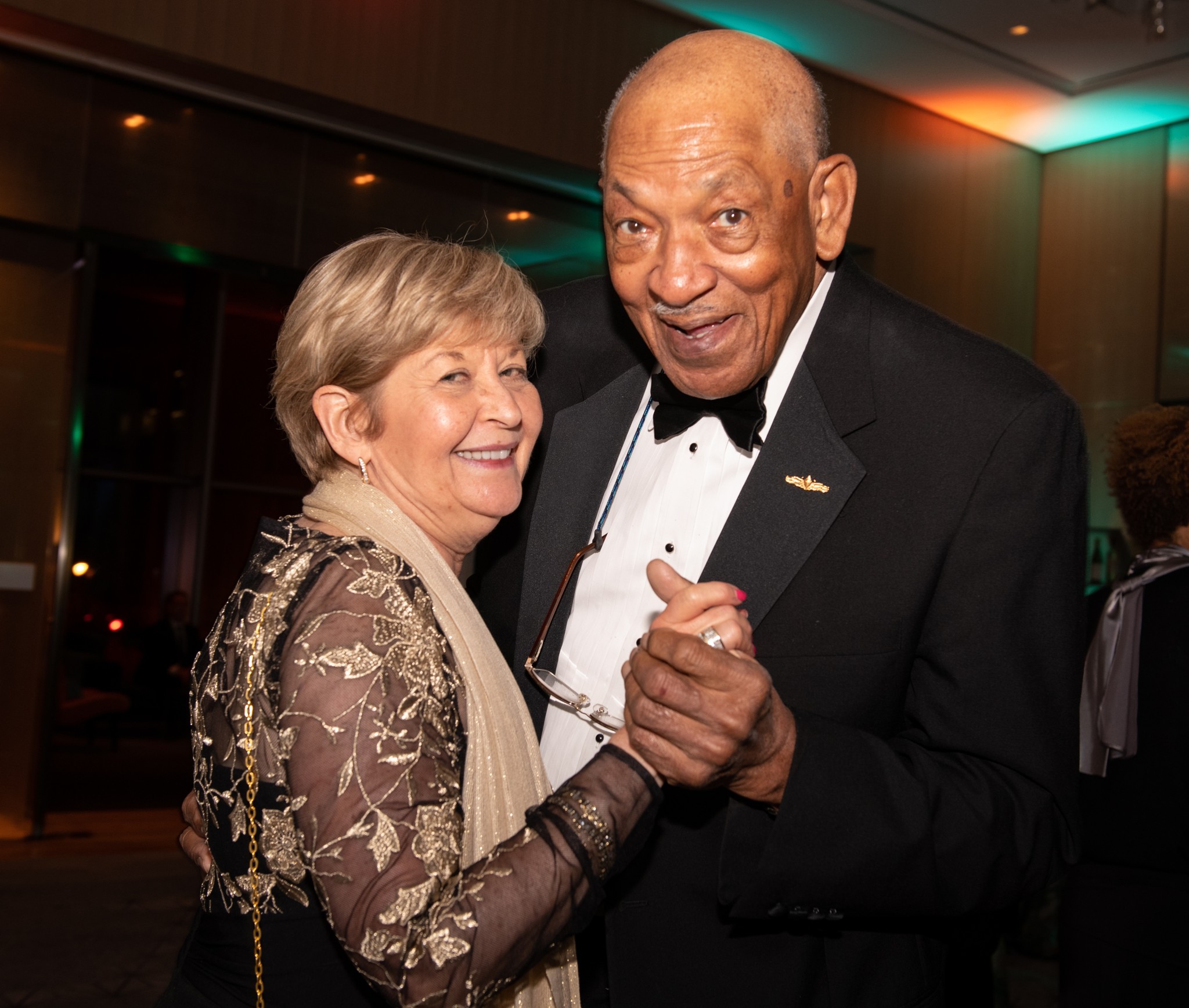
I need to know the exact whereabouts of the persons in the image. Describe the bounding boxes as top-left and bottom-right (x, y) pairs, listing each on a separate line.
(1088, 401), (1189, 1007)
(178, 27), (1088, 1008)
(159, 229), (755, 1007)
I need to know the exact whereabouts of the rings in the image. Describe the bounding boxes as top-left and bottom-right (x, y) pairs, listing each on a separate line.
(697, 625), (726, 650)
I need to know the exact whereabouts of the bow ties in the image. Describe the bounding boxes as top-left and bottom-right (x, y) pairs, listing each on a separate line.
(649, 371), (767, 454)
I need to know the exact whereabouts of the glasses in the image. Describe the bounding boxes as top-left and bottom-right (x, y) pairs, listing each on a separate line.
(522, 534), (626, 734)
